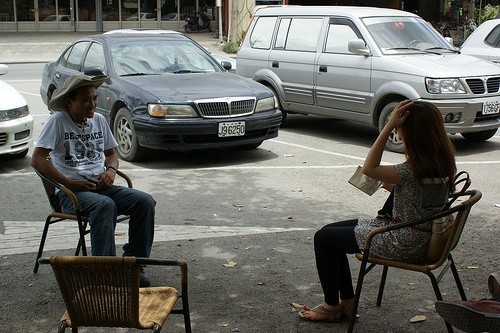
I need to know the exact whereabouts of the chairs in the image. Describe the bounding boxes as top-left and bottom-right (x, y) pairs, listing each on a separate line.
(347, 190), (482, 333)
(33, 167), (132, 273)
(38, 256), (192, 333)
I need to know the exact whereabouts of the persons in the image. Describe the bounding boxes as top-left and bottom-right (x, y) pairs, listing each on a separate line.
(30, 75), (156, 286)
(298, 99), (459, 323)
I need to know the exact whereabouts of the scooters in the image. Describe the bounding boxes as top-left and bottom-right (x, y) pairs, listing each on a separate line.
(184, 4), (213, 33)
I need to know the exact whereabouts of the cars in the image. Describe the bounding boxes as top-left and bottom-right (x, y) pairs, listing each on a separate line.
(39, 29), (282, 162)
(0, 64), (34, 158)
(460, 19), (500, 65)
(44, 15), (71, 21)
(103, 9), (214, 21)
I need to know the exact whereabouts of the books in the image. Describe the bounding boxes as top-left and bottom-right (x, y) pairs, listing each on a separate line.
(348, 166), (383, 196)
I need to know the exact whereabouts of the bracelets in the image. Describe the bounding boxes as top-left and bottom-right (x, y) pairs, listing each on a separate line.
(105, 166), (116, 172)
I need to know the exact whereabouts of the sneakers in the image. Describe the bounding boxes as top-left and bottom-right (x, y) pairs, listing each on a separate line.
(139, 266), (152, 287)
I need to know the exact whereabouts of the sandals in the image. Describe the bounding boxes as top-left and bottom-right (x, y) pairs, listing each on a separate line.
(299, 305), (360, 324)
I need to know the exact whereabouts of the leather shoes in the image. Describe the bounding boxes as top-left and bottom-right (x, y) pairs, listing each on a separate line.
(435, 298), (500, 333)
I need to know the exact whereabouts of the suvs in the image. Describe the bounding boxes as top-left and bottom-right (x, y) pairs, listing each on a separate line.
(236, 5), (500, 153)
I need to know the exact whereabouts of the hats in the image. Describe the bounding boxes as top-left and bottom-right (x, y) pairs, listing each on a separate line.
(49, 76), (111, 111)
